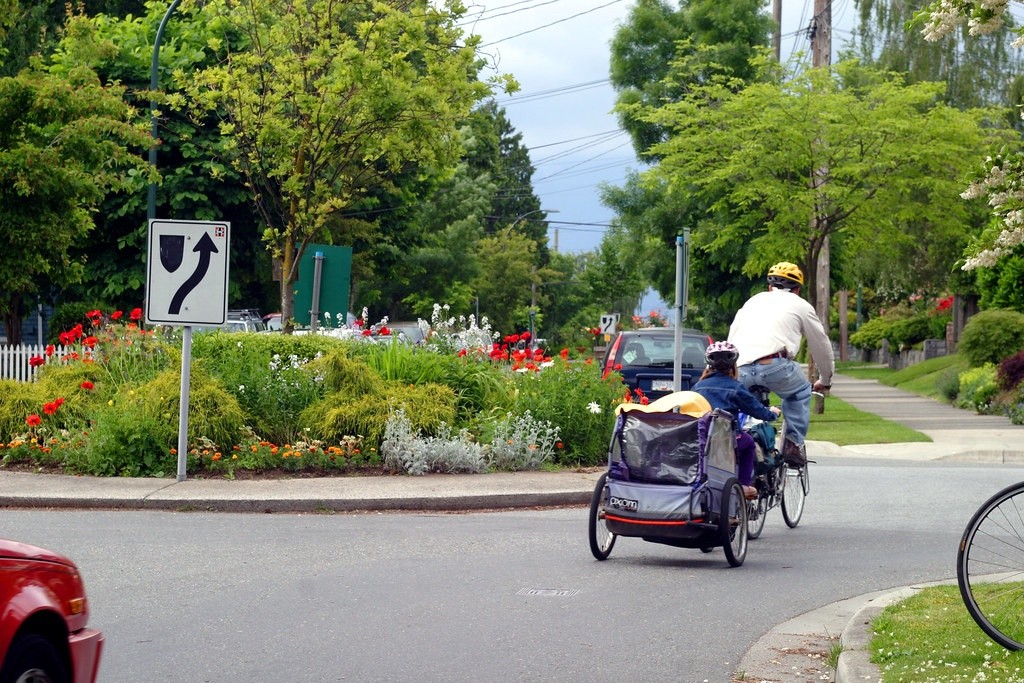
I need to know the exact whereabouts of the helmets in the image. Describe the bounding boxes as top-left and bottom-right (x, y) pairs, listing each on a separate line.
(767, 262), (804, 289)
(705, 341), (739, 366)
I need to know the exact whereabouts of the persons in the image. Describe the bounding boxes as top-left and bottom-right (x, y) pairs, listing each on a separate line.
(691, 340), (782, 499)
(699, 262), (835, 467)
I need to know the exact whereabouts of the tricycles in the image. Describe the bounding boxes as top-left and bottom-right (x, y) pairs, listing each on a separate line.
(582, 380), (781, 569)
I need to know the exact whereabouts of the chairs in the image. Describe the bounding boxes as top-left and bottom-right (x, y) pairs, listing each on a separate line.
(622, 343), (649, 366)
(682, 346), (706, 367)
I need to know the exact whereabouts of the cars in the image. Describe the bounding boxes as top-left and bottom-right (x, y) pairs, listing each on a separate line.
(0, 537), (105, 683)
(190, 307), (430, 350)
(601, 329), (716, 406)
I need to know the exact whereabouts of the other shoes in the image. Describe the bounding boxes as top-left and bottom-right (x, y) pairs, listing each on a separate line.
(742, 485), (757, 496)
(783, 439), (805, 469)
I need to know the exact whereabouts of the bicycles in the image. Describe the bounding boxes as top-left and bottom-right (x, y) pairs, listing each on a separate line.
(956, 479), (1024, 656)
(739, 382), (833, 541)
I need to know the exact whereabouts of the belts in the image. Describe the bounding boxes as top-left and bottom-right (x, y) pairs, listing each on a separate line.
(759, 352), (787, 360)
